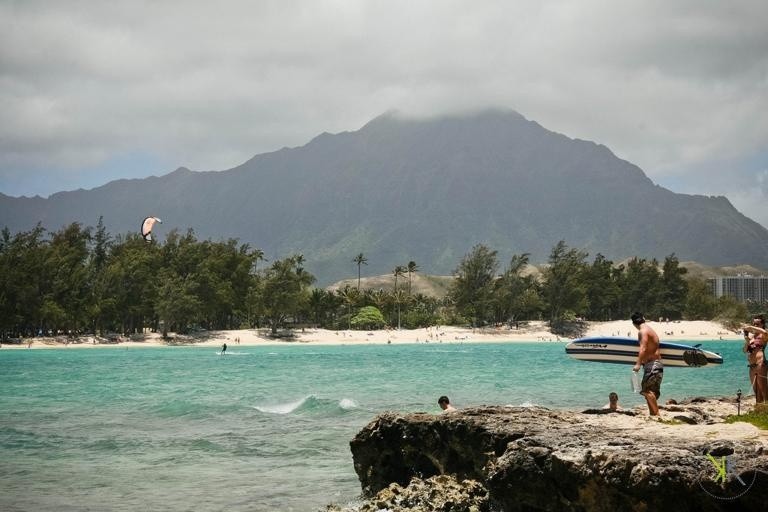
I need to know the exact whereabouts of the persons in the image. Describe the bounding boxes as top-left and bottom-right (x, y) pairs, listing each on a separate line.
(603, 392), (625, 411)
(436, 395), (457, 415)
(740, 314), (768, 405)
(630, 310), (664, 418)
(221, 342), (228, 355)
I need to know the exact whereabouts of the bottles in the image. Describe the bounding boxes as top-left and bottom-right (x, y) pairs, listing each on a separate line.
(630, 369), (642, 393)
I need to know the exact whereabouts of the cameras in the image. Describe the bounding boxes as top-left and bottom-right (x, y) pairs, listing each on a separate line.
(744, 331), (749, 336)
